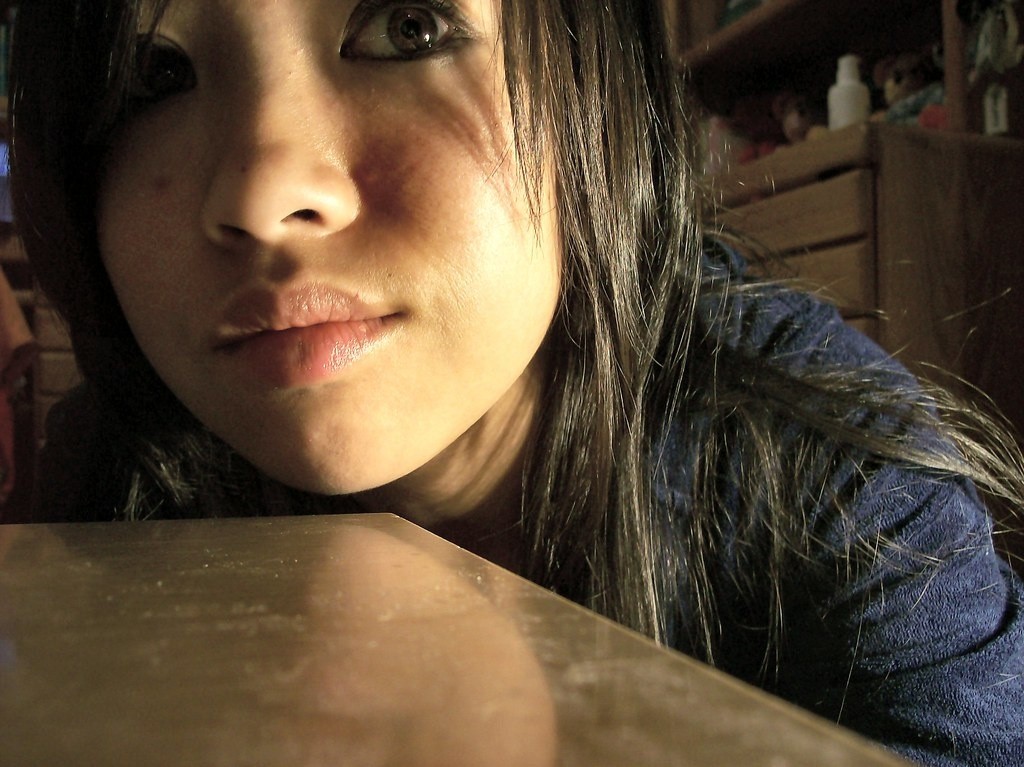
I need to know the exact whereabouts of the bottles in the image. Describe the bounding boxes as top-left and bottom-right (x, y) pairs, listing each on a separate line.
(829, 56), (868, 131)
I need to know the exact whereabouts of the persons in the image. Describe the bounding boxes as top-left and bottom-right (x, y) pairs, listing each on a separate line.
(0, 0), (1024, 767)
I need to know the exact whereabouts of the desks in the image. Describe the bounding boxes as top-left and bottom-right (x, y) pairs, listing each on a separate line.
(0, 513), (930, 767)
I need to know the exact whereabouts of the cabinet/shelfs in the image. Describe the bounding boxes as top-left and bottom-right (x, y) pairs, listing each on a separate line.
(660, 0), (1024, 593)
